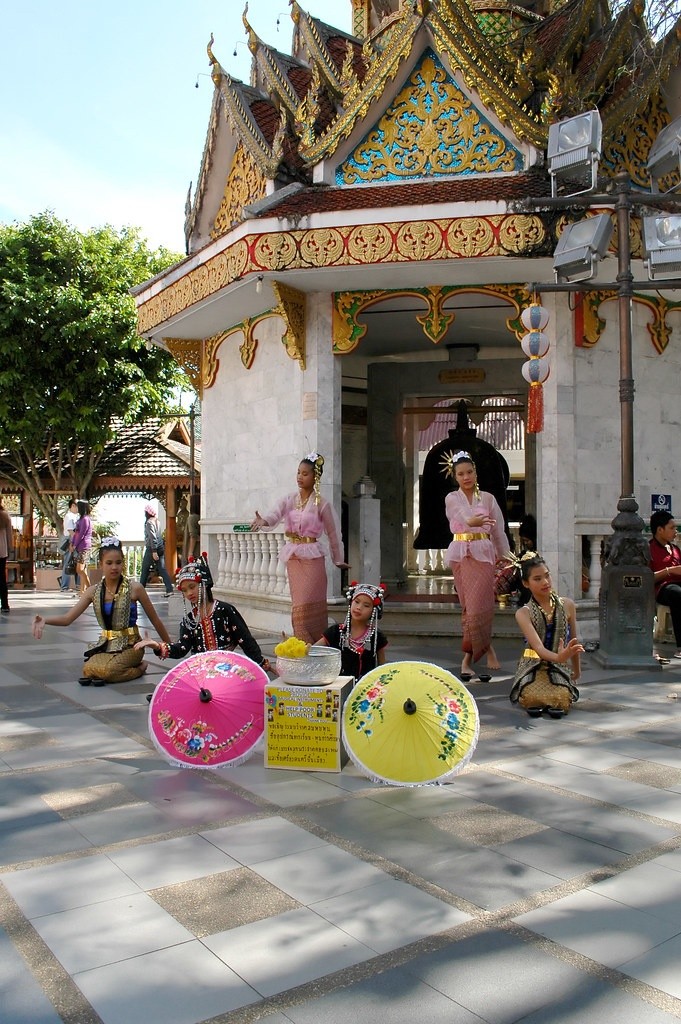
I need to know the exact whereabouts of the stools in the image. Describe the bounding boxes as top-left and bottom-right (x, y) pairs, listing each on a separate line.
(653, 605), (677, 643)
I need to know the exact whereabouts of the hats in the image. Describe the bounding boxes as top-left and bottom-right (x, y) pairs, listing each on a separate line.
(144, 505), (156, 517)
(341, 581), (386, 655)
(175, 553), (214, 630)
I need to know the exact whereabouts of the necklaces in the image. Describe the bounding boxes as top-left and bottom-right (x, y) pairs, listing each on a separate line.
(297, 493), (309, 508)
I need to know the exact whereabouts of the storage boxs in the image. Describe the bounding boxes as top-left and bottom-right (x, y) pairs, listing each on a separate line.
(263, 676), (354, 772)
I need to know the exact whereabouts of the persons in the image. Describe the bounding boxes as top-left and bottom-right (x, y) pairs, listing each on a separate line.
(0, 494), (13, 612)
(60, 498), (93, 597)
(312, 581), (388, 684)
(140, 505), (174, 597)
(646, 509), (681, 658)
(439, 450), (511, 677)
(493, 514), (536, 606)
(249, 448), (352, 644)
(133, 552), (280, 703)
(32, 537), (171, 683)
(503, 550), (586, 709)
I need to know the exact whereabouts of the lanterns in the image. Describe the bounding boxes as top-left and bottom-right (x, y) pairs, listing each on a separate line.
(521, 303), (551, 434)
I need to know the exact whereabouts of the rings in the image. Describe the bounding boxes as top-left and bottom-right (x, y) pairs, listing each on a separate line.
(157, 556), (158, 557)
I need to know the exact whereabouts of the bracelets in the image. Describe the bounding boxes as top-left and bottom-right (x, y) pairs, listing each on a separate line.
(666, 567), (671, 577)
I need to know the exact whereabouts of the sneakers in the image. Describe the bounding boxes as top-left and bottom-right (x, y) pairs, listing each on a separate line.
(160, 592), (173, 599)
(0, 607), (9, 615)
(60, 587), (73, 592)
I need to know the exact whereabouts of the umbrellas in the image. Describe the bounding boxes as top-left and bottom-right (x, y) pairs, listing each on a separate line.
(341, 661), (480, 788)
(148, 650), (270, 769)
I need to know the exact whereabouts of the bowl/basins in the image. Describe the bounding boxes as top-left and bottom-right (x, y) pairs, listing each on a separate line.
(276, 645), (342, 686)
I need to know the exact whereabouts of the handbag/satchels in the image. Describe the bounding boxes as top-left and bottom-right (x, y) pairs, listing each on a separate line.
(64, 552), (75, 575)
(59, 539), (69, 552)
